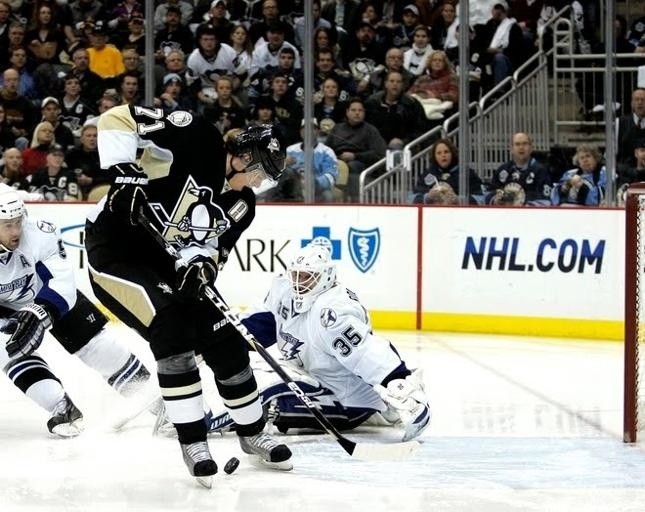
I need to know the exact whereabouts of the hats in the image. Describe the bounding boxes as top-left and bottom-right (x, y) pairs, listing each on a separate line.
(403, 4), (419, 15)
(88, 25), (105, 35)
(210, 0), (227, 9)
(41, 96), (59, 108)
(300, 117), (318, 128)
(163, 72), (182, 86)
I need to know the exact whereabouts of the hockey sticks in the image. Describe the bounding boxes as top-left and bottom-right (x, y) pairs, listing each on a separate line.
(134, 208), (425, 462)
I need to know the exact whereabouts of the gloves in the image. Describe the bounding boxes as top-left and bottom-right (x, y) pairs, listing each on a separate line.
(0, 300), (55, 361)
(105, 161), (150, 227)
(174, 245), (218, 299)
(374, 378), (431, 442)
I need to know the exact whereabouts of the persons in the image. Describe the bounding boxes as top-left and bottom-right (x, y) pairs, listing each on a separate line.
(617, 140), (645, 189)
(491, 133), (550, 199)
(0, 182), (170, 439)
(83, 98), (294, 489)
(0, 0), (524, 144)
(616, 88), (645, 147)
(205, 245), (430, 444)
(613, 15), (645, 53)
(31, 148), (80, 201)
(284, 117), (338, 203)
(551, 142), (615, 205)
(326, 102), (388, 204)
(405, 138), (490, 205)
(65, 124), (97, 174)
(22, 121), (54, 168)
(0, 147), (29, 190)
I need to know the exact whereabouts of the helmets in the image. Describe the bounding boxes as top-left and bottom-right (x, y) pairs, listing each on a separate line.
(0, 182), (28, 225)
(287, 243), (337, 314)
(225, 123), (286, 195)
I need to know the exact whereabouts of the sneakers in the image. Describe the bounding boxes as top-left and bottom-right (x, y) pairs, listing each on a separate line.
(180, 440), (218, 477)
(47, 393), (83, 432)
(240, 430), (292, 463)
